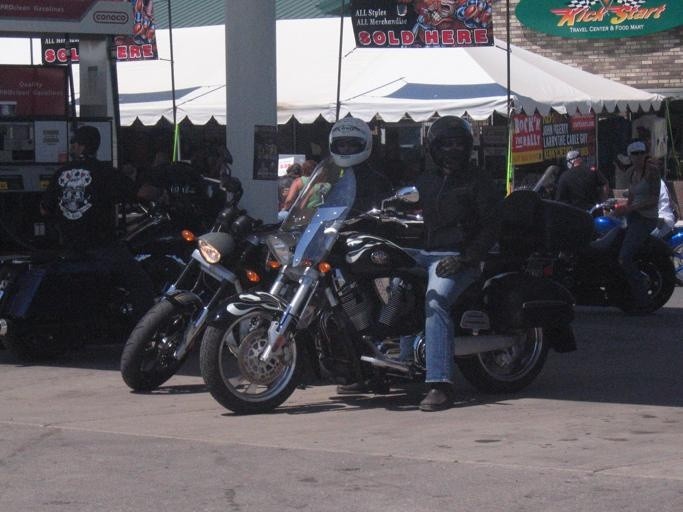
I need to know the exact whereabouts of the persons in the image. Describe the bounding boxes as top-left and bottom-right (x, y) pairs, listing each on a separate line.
(337, 115), (501, 411)
(44, 127), (227, 360)
(276, 116), (421, 219)
(613, 142), (661, 314)
(556, 150), (610, 221)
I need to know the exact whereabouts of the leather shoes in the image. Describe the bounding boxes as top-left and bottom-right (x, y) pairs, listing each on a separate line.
(336, 376), (390, 395)
(420, 384), (452, 411)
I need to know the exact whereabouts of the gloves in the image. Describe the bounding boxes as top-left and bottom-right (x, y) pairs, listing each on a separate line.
(435, 256), (461, 278)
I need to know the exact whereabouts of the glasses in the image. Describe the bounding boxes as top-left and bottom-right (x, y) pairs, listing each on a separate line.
(630, 151), (647, 155)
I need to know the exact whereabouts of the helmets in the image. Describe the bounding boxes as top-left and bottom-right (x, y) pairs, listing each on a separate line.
(69, 126), (101, 160)
(567, 151), (582, 170)
(328, 117), (373, 167)
(427, 116), (473, 168)
(628, 141), (646, 155)
